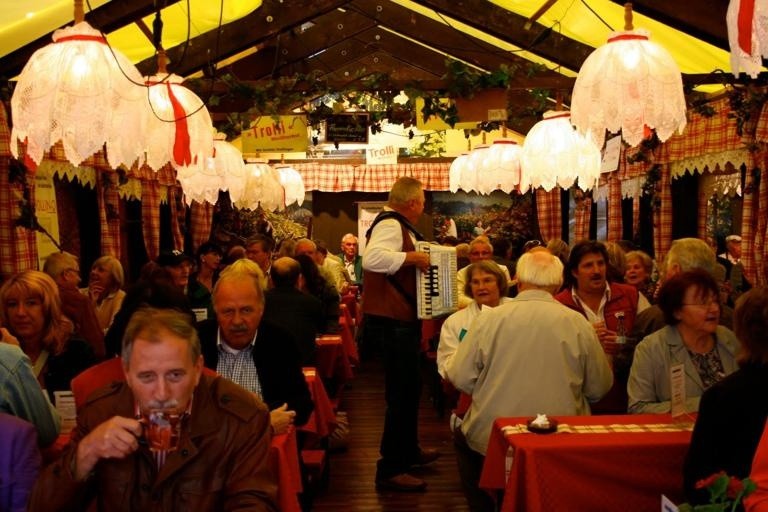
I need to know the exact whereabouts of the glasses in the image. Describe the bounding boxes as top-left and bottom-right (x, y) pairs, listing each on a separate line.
(69, 268), (81, 275)
(682, 297), (718, 308)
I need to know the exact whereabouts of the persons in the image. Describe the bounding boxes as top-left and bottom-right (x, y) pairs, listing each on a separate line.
(474, 219), (491, 236)
(354, 175), (474, 493)
(1, 229), (362, 511)
(431, 229), (767, 511)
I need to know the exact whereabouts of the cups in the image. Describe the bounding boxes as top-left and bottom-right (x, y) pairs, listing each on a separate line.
(145, 402), (181, 454)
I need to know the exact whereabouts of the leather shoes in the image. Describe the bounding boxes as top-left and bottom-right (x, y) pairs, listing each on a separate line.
(380, 473), (427, 491)
(415, 447), (442, 465)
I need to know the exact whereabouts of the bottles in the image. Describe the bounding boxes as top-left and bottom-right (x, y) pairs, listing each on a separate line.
(616, 315), (628, 348)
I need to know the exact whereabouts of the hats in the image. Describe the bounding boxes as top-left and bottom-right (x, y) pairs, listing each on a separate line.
(159, 250), (192, 266)
(725, 235), (741, 242)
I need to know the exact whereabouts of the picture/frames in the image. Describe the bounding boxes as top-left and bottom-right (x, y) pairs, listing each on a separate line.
(324, 112), (370, 145)
(357, 198), (390, 257)
